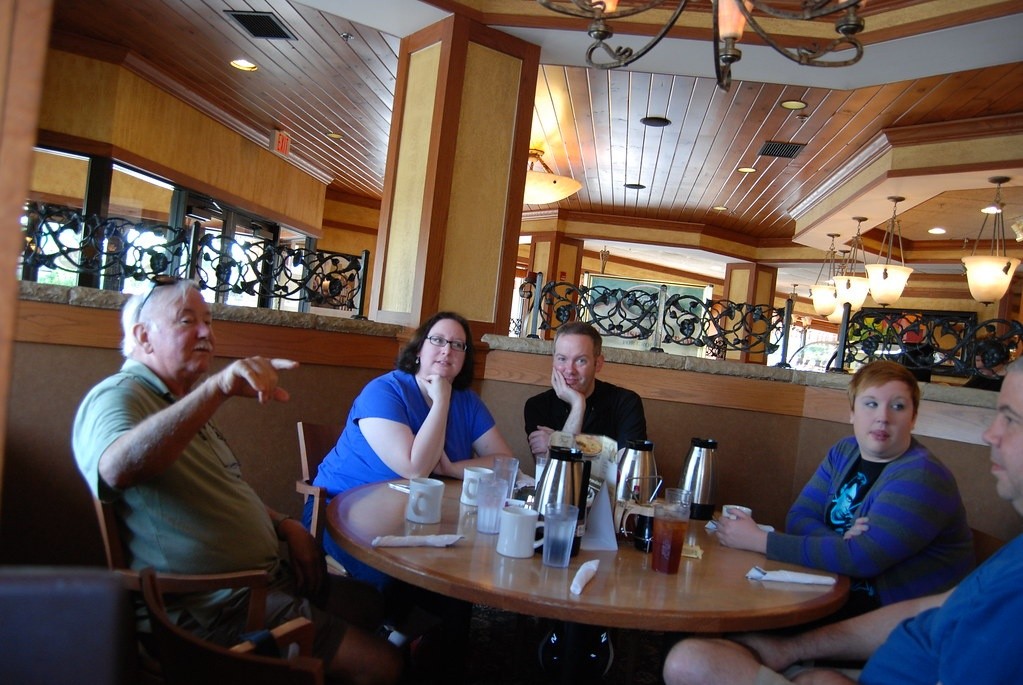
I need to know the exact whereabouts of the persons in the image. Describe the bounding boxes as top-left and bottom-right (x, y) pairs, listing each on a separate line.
(300, 310), (522, 652)
(717, 361), (969, 625)
(71, 275), (407, 685)
(663, 354), (1023, 685)
(523, 321), (648, 673)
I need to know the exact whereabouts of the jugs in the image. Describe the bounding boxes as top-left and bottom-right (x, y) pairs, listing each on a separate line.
(527, 444), (592, 556)
(613, 439), (659, 542)
(677, 437), (719, 520)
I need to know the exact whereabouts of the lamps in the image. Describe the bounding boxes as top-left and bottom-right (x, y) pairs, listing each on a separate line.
(538, 0), (866, 92)
(810, 196), (913, 325)
(962, 176), (1021, 306)
(523, 149), (582, 205)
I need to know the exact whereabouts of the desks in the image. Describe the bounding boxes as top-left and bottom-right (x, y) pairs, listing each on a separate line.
(327, 477), (850, 685)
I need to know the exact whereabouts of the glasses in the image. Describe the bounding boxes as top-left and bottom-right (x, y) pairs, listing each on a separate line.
(425, 335), (467, 353)
(136, 275), (202, 325)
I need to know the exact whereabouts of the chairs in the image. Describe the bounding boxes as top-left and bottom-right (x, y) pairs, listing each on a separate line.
(94, 421), (474, 684)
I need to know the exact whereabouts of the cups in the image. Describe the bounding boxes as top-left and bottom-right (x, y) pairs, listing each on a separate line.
(666, 487), (693, 511)
(542, 502), (579, 569)
(496, 506), (544, 559)
(533, 453), (549, 492)
(476, 477), (508, 534)
(406, 477), (444, 524)
(494, 457), (519, 499)
(722, 504), (753, 520)
(653, 504), (689, 574)
(460, 468), (494, 505)
(622, 504), (656, 552)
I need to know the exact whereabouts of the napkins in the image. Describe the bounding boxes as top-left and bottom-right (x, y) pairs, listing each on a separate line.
(372, 534), (466, 547)
(705, 520), (775, 532)
(745, 565), (836, 586)
(570, 559), (600, 595)
